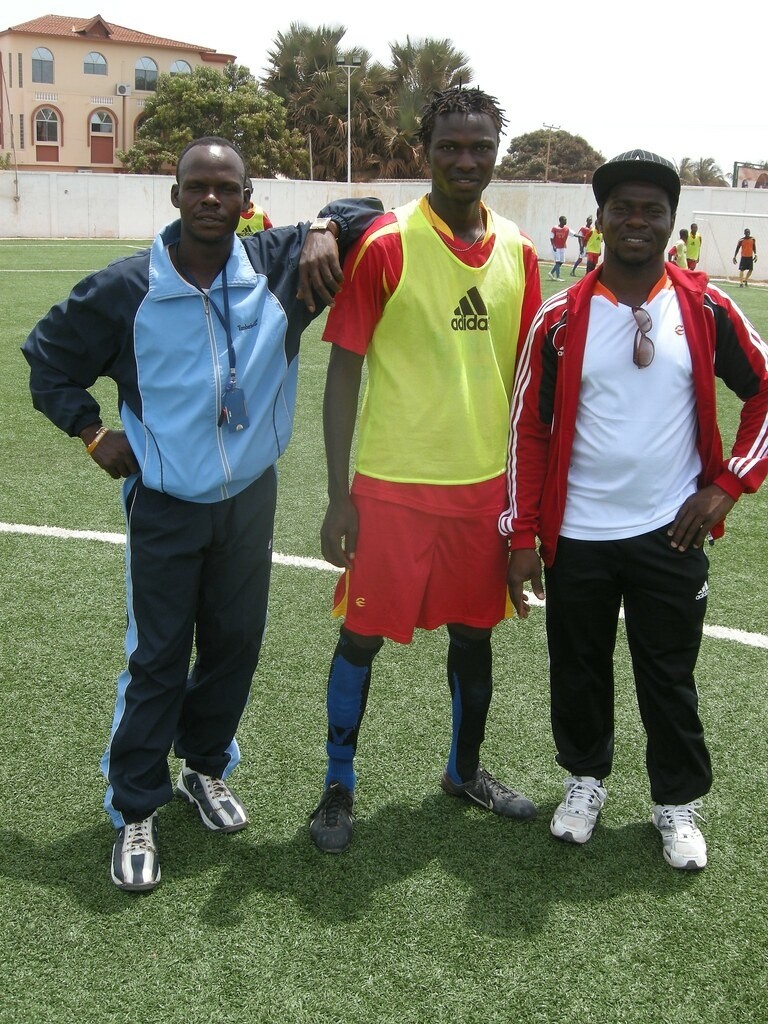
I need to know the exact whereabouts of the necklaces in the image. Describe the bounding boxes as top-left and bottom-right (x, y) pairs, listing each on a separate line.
(427, 194), (484, 252)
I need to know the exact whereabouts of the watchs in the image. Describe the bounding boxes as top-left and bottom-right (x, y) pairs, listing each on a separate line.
(309, 217), (339, 240)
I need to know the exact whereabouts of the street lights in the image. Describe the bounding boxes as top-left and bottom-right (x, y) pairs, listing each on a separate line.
(335, 54), (361, 182)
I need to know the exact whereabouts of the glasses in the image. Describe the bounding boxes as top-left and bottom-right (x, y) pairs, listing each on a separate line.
(632, 306), (655, 370)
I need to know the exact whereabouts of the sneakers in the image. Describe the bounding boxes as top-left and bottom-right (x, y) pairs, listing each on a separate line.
(308, 780), (354, 853)
(175, 759), (248, 833)
(111, 810), (161, 891)
(440, 762), (537, 819)
(550, 777), (607, 843)
(651, 799), (707, 868)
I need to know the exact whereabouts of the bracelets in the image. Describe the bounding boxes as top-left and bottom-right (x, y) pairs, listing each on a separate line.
(87, 427), (110, 455)
(754, 254), (757, 255)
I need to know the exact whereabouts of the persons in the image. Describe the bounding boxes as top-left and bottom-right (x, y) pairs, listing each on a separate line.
(583, 219), (604, 274)
(686, 223), (702, 270)
(570, 217), (594, 277)
(234, 176), (273, 238)
(496, 148), (768, 870)
(547, 216), (585, 282)
(733, 228), (757, 288)
(667, 229), (689, 270)
(309, 83), (543, 854)
(21, 136), (384, 891)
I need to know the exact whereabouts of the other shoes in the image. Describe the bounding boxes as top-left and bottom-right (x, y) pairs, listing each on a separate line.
(570, 272), (576, 277)
(548, 272), (556, 280)
(740, 283), (744, 288)
(556, 278), (565, 282)
(743, 280), (749, 288)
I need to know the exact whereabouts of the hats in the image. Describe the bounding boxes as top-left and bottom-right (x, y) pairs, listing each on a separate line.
(592, 150), (680, 209)
